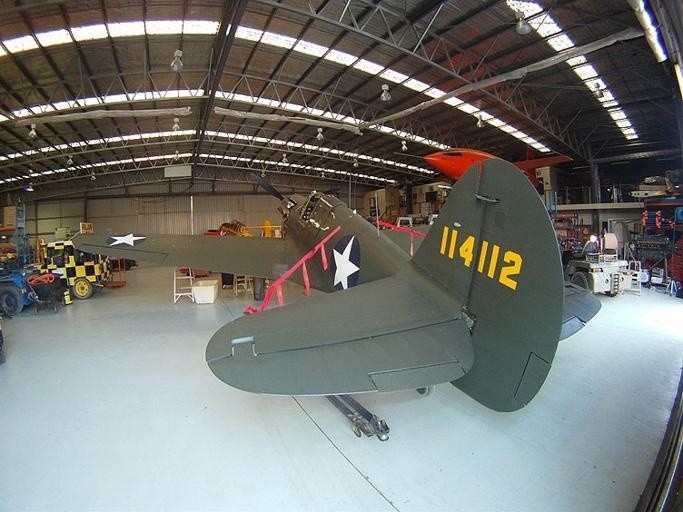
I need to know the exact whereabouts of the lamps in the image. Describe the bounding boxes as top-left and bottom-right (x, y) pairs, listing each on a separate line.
(380, 84), (392, 101)
(516, 10), (533, 35)
(475, 115), (485, 129)
(316, 128), (325, 140)
(400, 141), (408, 151)
(171, 51), (185, 70)
(28, 124), (38, 138)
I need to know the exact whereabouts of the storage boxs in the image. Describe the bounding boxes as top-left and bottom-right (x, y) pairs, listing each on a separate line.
(191, 279), (219, 304)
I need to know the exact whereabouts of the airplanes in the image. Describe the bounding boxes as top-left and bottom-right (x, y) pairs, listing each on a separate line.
(392, 146), (575, 188)
(70, 158), (602, 443)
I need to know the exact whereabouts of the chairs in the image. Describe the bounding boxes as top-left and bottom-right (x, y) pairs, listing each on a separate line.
(628, 261), (642, 296)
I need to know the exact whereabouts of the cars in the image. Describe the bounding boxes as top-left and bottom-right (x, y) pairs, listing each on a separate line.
(0, 239), (139, 317)
(562, 232), (633, 297)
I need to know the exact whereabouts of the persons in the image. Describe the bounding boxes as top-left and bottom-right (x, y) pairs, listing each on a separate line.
(581, 233), (597, 252)
